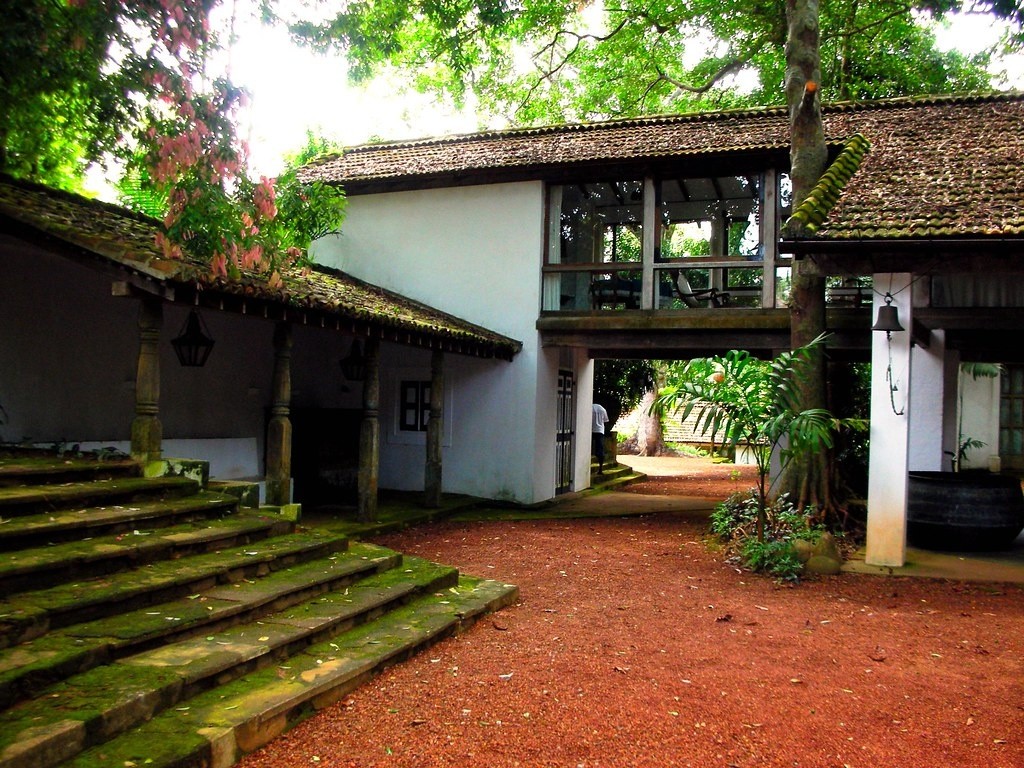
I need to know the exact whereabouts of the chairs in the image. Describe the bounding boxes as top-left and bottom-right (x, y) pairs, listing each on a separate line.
(671, 269), (736, 309)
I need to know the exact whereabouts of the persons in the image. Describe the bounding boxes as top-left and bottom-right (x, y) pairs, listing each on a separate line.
(591, 394), (609, 476)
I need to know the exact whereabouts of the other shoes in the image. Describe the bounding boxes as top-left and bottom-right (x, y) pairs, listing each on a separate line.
(597, 471), (604, 475)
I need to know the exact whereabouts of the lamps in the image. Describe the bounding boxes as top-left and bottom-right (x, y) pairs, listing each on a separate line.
(171, 309), (215, 366)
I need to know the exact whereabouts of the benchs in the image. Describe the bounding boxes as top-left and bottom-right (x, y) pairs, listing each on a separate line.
(591, 280), (640, 309)
(672, 285), (764, 297)
